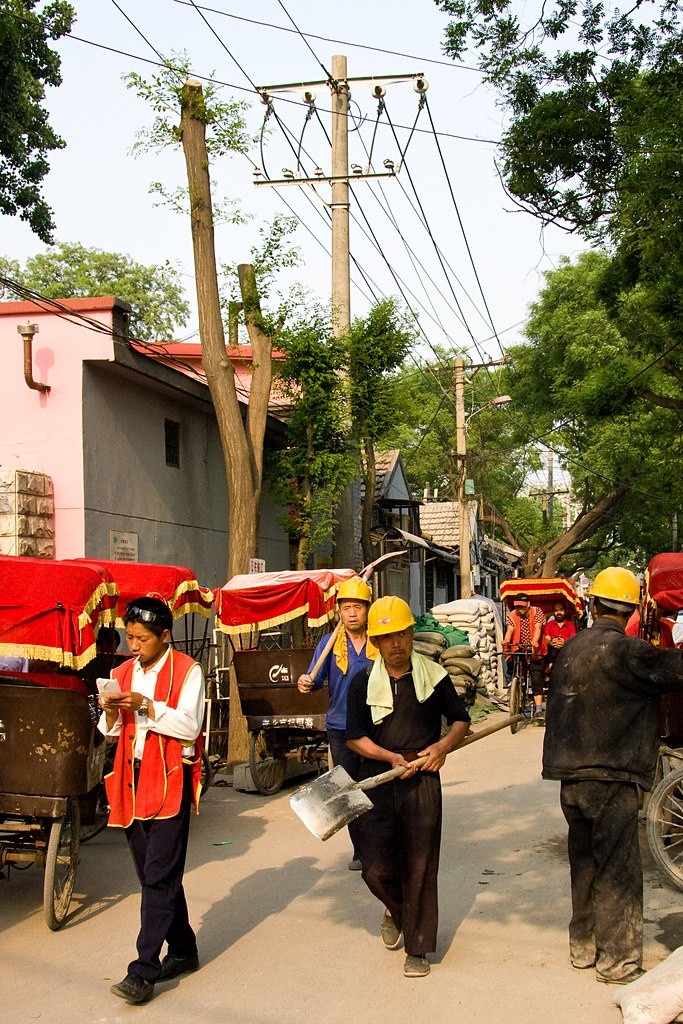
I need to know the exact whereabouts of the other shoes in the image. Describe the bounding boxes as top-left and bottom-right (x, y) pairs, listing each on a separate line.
(571, 961), (596, 969)
(507, 686), (511, 696)
(403, 953), (430, 976)
(535, 706), (542, 716)
(595, 967), (647, 984)
(381, 908), (402, 948)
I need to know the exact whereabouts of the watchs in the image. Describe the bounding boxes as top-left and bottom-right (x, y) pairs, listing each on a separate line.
(137, 696), (149, 716)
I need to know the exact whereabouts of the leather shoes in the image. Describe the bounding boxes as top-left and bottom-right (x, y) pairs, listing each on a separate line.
(110, 973), (154, 1001)
(155, 954), (199, 983)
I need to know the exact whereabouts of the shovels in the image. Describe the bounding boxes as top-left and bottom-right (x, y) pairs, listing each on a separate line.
(288, 713), (527, 842)
(299, 551), (408, 682)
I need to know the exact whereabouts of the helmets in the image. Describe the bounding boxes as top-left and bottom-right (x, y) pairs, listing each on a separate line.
(367, 596), (417, 636)
(336, 579), (371, 602)
(589, 567), (640, 605)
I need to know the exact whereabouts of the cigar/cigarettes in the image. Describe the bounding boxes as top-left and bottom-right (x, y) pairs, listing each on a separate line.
(132, 655), (141, 664)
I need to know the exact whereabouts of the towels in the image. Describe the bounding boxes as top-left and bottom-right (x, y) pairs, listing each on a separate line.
(366, 648), (448, 725)
(333, 623), (379, 675)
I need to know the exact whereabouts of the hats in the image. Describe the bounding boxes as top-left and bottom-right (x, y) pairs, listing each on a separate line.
(514, 593), (528, 606)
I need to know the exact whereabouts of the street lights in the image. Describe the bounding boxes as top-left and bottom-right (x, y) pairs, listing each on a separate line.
(456, 394), (512, 598)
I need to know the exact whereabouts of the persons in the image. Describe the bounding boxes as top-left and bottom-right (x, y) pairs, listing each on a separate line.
(541, 566), (683, 985)
(345, 596), (471, 978)
(97, 596), (206, 1001)
(96, 627), (121, 654)
(500, 593), (576, 717)
(297, 577), (380, 870)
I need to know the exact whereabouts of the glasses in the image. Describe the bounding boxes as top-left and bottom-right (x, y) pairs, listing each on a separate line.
(126, 606), (156, 623)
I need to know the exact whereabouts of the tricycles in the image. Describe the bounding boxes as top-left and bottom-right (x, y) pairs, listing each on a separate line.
(66, 556), (215, 802)
(498, 578), (588, 734)
(639, 552), (683, 894)
(212, 568), (357, 795)
(1, 555), (119, 933)
